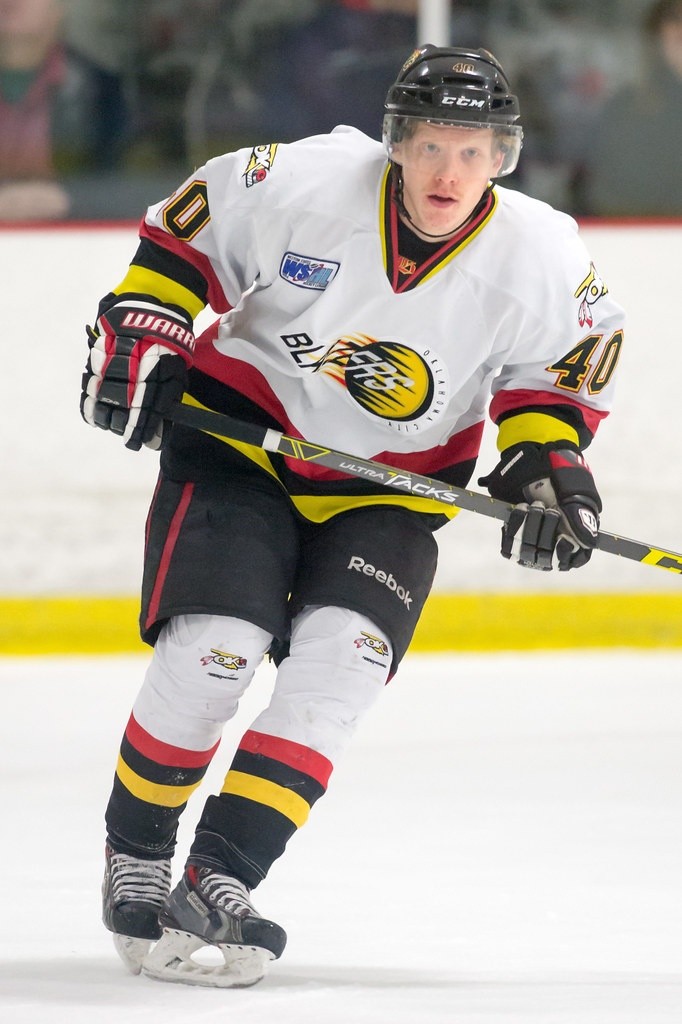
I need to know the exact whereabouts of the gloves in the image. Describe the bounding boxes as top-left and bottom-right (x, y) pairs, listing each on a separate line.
(77, 289), (198, 452)
(479, 404), (601, 575)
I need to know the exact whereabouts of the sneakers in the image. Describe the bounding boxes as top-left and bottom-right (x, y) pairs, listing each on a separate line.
(99, 832), (175, 977)
(161, 852), (284, 989)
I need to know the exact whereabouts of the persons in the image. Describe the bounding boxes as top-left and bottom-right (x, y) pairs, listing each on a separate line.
(78, 43), (621, 990)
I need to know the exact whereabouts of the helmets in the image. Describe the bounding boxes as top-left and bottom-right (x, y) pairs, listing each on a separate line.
(383, 43), (520, 153)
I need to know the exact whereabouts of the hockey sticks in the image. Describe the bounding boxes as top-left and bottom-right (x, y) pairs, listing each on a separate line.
(163, 402), (681, 578)
